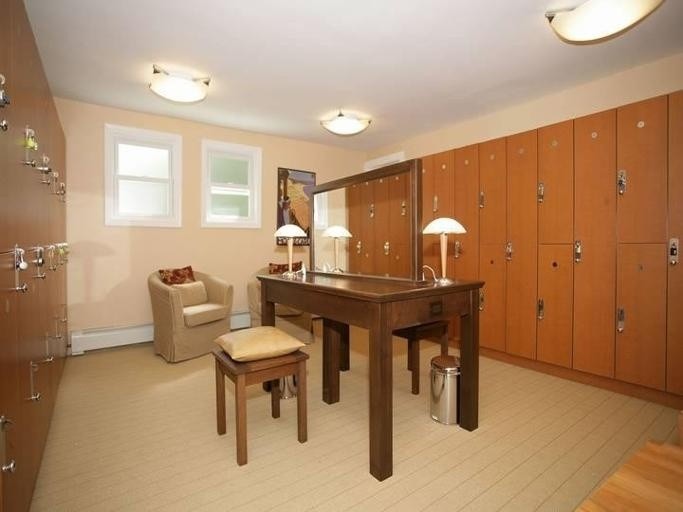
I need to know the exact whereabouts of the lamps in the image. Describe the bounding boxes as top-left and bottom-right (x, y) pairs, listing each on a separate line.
(148, 63), (212, 105)
(274, 224), (307, 271)
(422, 217), (467, 287)
(545, 0), (664, 45)
(320, 225), (354, 274)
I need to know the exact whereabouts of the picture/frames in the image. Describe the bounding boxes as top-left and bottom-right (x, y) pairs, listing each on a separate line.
(277, 167), (316, 246)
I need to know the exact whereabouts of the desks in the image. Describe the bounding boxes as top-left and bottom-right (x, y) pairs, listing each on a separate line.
(256, 271), (485, 482)
(573, 438), (683, 512)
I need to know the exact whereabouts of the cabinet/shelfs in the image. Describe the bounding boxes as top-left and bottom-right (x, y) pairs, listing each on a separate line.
(453, 144), (479, 355)
(346, 174), (359, 274)
(616, 96), (667, 244)
(573, 107), (617, 389)
(389, 158), (420, 337)
(538, 117), (574, 244)
(479, 136), (506, 245)
(615, 243), (667, 406)
(431, 243), (453, 350)
(359, 170), (374, 276)
(374, 166), (390, 279)
(506, 128), (538, 378)
(416, 154), (433, 350)
(536, 243), (573, 383)
(668, 90), (683, 412)
(0, 0), (68, 512)
(479, 245), (506, 363)
(432, 150), (454, 245)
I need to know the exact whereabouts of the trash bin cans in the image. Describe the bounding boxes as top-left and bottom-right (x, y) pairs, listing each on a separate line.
(278, 373), (298, 400)
(429, 355), (461, 425)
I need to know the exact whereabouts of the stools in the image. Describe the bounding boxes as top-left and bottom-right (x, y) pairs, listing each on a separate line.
(210, 346), (309, 466)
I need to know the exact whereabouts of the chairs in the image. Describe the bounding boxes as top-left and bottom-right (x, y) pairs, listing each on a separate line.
(246, 266), (304, 327)
(147, 270), (233, 363)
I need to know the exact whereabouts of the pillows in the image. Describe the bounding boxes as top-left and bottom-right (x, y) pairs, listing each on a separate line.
(159, 265), (195, 286)
(269, 261), (302, 273)
(172, 281), (208, 306)
(214, 325), (306, 362)
